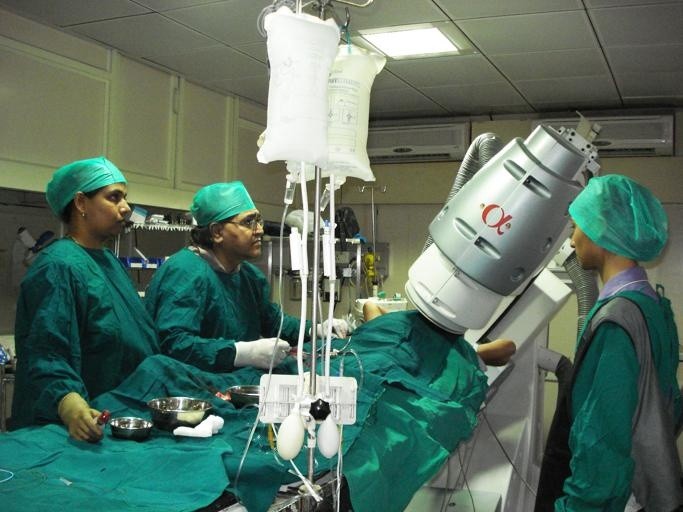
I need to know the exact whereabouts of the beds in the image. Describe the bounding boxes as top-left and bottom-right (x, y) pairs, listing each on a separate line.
(0, 337), (515, 511)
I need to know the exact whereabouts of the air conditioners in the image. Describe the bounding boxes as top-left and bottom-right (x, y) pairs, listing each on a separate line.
(366, 122), (471, 161)
(531, 116), (674, 159)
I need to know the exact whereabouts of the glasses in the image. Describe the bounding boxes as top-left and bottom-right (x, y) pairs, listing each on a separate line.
(223, 217), (267, 230)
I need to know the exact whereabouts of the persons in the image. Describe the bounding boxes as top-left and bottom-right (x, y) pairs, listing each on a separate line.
(9, 157), (163, 445)
(362, 299), (515, 374)
(533, 173), (683, 512)
(143, 179), (352, 374)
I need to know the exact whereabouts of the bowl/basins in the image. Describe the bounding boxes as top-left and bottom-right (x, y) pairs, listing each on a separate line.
(147, 396), (212, 432)
(225, 385), (259, 409)
(109, 416), (153, 442)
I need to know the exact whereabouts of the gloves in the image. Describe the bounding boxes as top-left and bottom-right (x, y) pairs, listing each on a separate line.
(58, 392), (102, 442)
(309, 317), (349, 339)
(234, 337), (292, 370)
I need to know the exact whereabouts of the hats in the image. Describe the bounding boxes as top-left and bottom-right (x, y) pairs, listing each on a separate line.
(569, 174), (669, 261)
(46, 157), (127, 217)
(191, 181), (256, 227)
